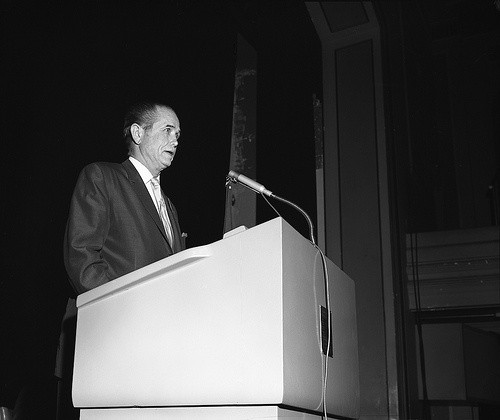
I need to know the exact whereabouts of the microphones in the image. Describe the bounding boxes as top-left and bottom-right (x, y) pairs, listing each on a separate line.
(228, 170), (315, 244)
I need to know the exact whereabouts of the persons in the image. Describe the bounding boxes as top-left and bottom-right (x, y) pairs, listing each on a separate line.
(55, 102), (187, 420)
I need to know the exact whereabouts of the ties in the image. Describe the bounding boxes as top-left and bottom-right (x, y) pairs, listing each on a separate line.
(149, 178), (174, 247)
